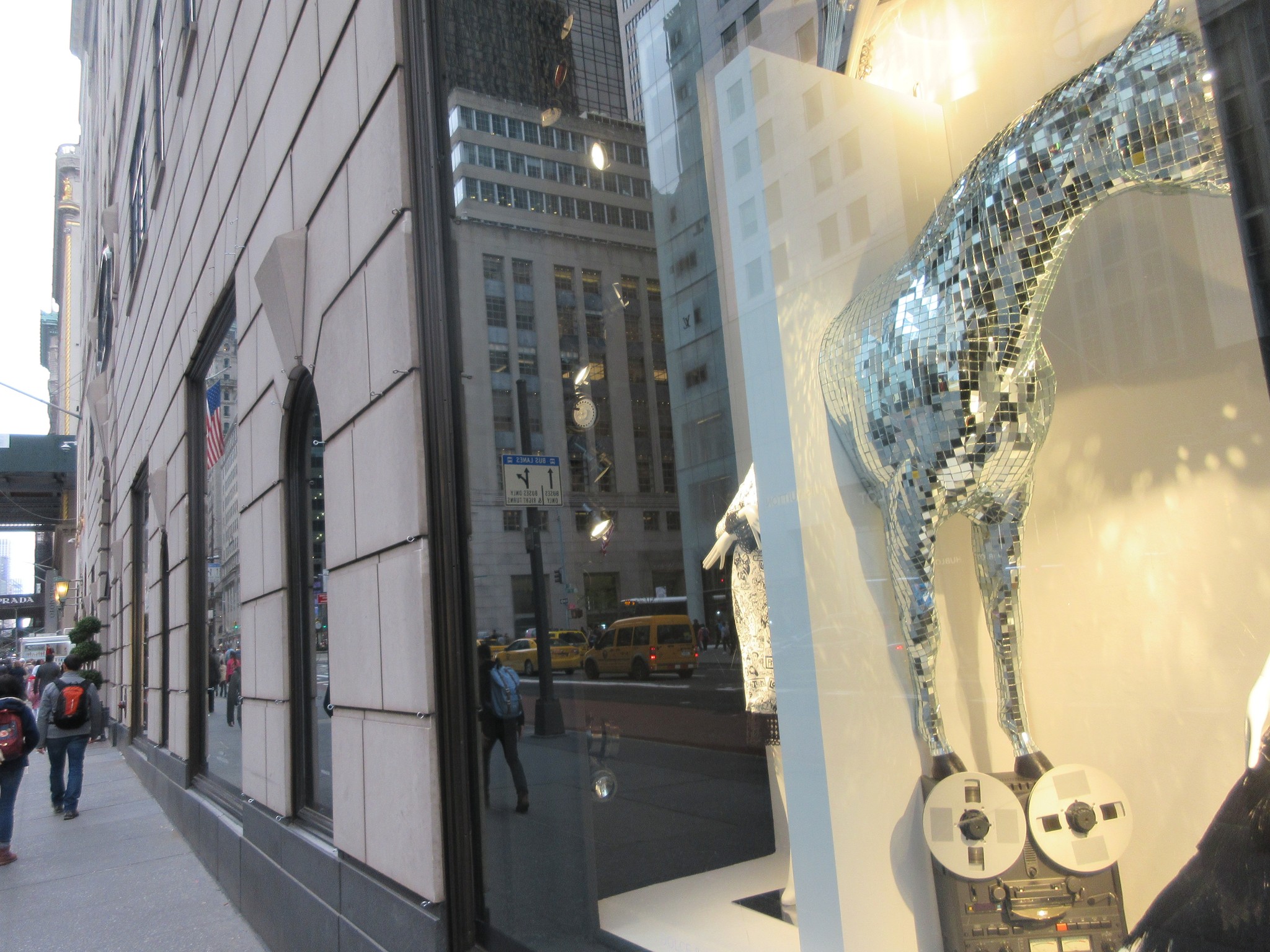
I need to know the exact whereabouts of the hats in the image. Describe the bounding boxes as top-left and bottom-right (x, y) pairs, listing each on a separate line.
(19, 658), (25, 662)
(14, 661), (20, 667)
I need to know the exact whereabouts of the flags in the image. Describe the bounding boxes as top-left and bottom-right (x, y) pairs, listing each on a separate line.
(206, 379), (225, 470)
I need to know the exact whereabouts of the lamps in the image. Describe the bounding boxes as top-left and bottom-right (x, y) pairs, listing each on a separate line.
(535, 7), (611, 172)
(61, 441), (77, 452)
(54, 587), (79, 610)
(55, 575), (83, 605)
(68, 538), (76, 543)
(568, 435), (611, 482)
(582, 500), (613, 542)
(562, 357), (591, 390)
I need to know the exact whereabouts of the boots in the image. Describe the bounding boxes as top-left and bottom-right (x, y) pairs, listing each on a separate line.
(0, 845), (18, 865)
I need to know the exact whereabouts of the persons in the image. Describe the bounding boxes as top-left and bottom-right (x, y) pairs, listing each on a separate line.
(0, 674), (40, 865)
(478, 644), (532, 815)
(693, 618), (742, 657)
(206, 644), (242, 731)
(490, 629), (510, 646)
(0, 653), (61, 721)
(37, 654), (100, 821)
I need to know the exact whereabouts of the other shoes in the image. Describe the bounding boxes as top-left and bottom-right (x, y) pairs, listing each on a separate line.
(514, 794), (530, 813)
(209, 711), (214, 713)
(215, 693), (218, 697)
(54, 805), (63, 814)
(63, 810), (80, 820)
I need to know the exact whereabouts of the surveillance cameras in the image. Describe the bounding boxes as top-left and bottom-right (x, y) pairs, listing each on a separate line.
(68, 540), (73, 544)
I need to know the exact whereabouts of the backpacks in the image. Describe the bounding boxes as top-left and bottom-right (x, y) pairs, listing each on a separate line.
(482, 658), (523, 719)
(0, 708), (23, 765)
(47, 679), (91, 729)
(233, 666), (243, 705)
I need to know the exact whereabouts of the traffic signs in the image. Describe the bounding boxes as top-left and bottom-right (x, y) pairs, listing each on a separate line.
(503, 455), (564, 507)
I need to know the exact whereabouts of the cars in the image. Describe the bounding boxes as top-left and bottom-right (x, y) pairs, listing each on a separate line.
(475, 639), (582, 678)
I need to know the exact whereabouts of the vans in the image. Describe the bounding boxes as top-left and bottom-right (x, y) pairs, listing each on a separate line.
(582, 613), (700, 682)
(547, 631), (590, 655)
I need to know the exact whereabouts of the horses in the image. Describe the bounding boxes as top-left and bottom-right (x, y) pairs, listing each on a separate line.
(817, 0), (1231, 782)
